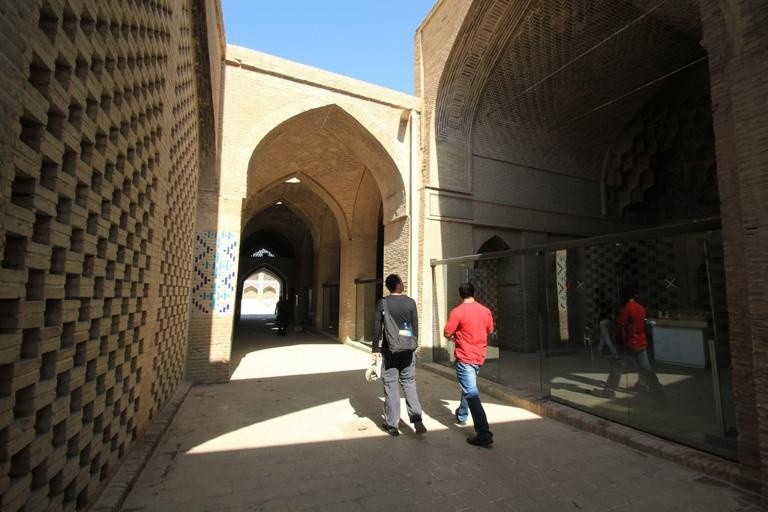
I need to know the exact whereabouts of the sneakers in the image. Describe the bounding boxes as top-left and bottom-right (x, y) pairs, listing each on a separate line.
(383, 424), (399, 436)
(456, 409), (462, 424)
(415, 420), (427, 434)
(467, 432), (493, 445)
(594, 387), (615, 398)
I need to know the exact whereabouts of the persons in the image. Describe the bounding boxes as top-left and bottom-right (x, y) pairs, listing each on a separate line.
(369, 273), (429, 438)
(591, 285), (664, 399)
(443, 281), (497, 447)
(273, 295), (290, 337)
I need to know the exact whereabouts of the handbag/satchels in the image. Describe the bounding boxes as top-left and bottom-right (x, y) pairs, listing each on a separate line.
(384, 313), (417, 354)
